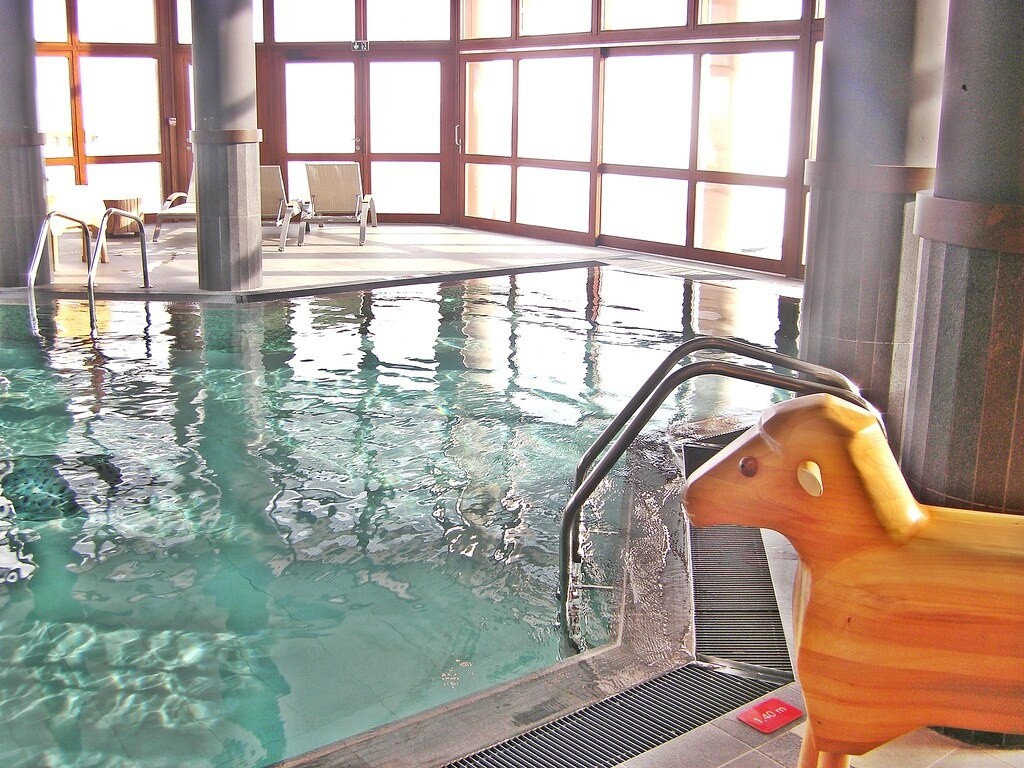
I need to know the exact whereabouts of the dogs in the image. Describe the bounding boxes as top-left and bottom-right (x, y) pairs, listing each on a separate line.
(682, 391), (1024, 768)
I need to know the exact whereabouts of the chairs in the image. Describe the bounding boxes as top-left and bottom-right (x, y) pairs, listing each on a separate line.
(297, 164), (377, 248)
(261, 165), (311, 252)
(152, 163), (196, 243)
